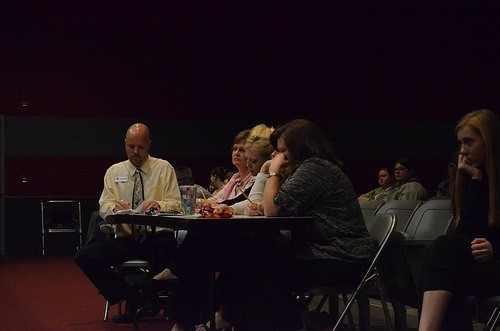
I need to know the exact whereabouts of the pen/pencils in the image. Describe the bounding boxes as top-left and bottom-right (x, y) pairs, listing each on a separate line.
(120, 199), (124, 202)
(200, 188), (212, 212)
(239, 187), (263, 216)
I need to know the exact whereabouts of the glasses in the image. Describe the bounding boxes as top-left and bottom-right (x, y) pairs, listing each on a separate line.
(232, 147), (245, 153)
(394, 167), (407, 172)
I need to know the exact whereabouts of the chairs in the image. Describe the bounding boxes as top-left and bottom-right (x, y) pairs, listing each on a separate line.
(99, 224), (149, 322)
(291, 199), (500, 331)
(39, 199), (83, 257)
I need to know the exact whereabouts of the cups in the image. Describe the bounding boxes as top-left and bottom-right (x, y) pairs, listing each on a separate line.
(179, 186), (197, 215)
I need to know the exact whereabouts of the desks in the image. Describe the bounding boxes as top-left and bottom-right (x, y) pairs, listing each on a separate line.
(115, 214), (316, 331)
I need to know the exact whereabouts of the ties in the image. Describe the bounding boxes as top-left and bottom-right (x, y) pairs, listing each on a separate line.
(132, 168), (144, 210)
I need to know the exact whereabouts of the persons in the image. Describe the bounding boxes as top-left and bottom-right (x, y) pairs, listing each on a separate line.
(358, 110), (500, 331)
(73, 124), (183, 323)
(151, 119), (374, 331)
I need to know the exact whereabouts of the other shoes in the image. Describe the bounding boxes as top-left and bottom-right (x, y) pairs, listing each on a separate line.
(136, 306), (162, 318)
(112, 310), (138, 323)
(160, 320), (235, 331)
(140, 279), (180, 291)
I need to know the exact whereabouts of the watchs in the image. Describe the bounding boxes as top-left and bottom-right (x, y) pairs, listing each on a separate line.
(266, 171), (282, 178)
